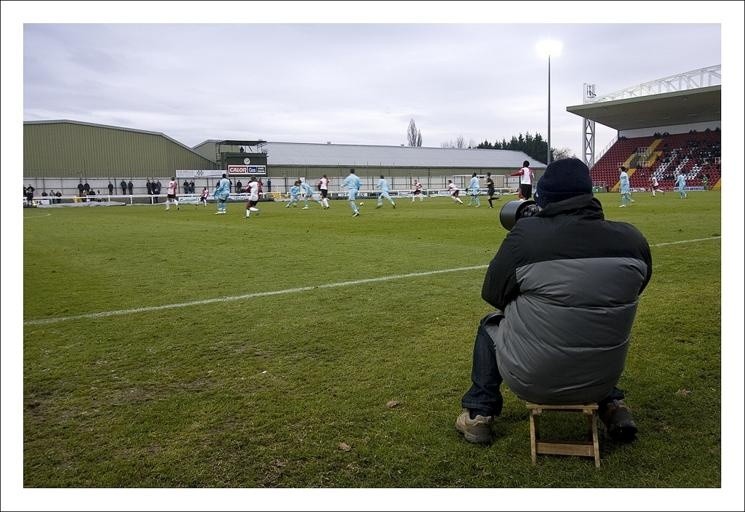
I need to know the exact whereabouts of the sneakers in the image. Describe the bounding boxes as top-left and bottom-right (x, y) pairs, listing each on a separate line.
(604, 399), (638, 443)
(456, 408), (493, 443)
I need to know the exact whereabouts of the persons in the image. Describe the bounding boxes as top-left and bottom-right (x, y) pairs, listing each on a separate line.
(375, 175), (396, 209)
(510, 161), (534, 200)
(448, 180), (464, 204)
(484, 173), (499, 208)
(24, 169), (330, 218)
(341, 168), (362, 217)
(649, 127), (721, 199)
(618, 166), (635, 208)
(412, 180), (423, 202)
(467, 172), (481, 207)
(455, 158), (653, 445)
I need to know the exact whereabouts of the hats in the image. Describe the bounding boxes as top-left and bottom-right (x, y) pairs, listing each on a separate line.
(534, 158), (593, 209)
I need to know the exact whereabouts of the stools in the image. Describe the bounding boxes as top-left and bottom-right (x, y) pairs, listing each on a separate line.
(527, 399), (601, 469)
